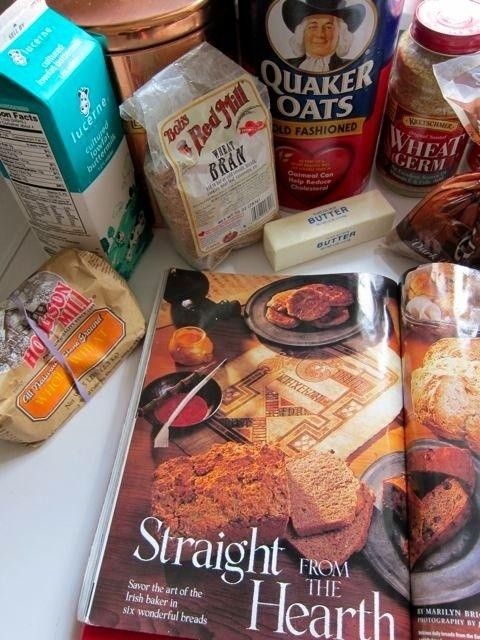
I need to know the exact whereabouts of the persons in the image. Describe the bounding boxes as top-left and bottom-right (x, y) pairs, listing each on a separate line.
(282, 0), (367, 76)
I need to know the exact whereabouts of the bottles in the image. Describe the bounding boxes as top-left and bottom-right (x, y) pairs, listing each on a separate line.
(375, 2), (480, 201)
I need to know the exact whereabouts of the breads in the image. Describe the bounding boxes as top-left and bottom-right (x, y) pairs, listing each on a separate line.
(146, 264), (480, 574)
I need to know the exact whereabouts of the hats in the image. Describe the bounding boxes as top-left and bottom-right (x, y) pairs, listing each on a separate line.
(282, 0), (367, 33)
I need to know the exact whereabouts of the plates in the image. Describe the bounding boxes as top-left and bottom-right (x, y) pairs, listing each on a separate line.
(141, 371), (225, 427)
(355, 438), (479, 610)
(245, 275), (377, 348)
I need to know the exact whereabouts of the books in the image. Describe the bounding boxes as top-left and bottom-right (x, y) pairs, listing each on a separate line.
(74, 254), (480, 639)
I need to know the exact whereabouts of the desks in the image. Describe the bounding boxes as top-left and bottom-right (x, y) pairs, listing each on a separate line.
(1, 123), (475, 635)
